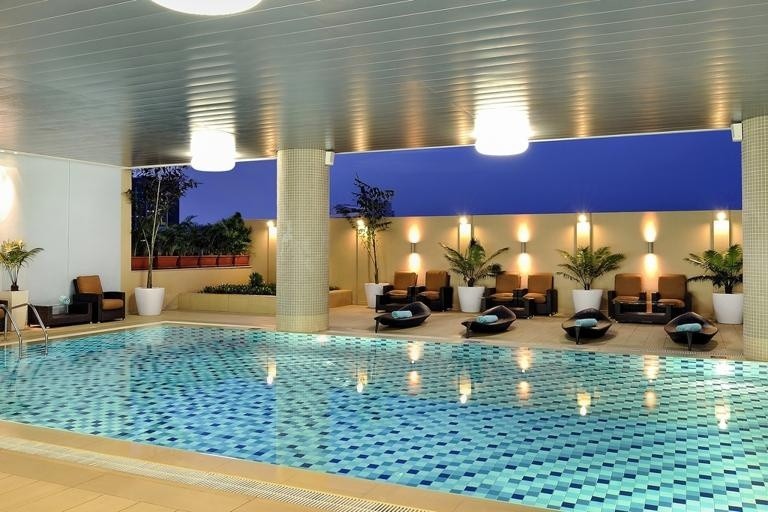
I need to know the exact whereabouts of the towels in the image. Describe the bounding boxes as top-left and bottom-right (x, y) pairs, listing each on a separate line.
(676, 323), (702, 332)
(59, 295), (71, 305)
(476, 315), (498, 323)
(392, 311), (413, 319)
(576, 318), (598, 327)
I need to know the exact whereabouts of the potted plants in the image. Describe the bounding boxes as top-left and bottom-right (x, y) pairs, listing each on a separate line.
(122, 214), (253, 271)
(0, 238), (46, 332)
(437, 238), (509, 313)
(554, 244), (625, 314)
(130, 165), (204, 316)
(682, 243), (743, 325)
(332, 173), (395, 308)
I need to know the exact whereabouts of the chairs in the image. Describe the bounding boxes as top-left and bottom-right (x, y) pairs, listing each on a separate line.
(664, 311), (719, 351)
(486, 273), (558, 318)
(374, 302), (431, 333)
(461, 305), (517, 338)
(383, 270), (453, 312)
(605, 273), (693, 318)
(561, 308), (613, 344)
(72, 275), (125, 324)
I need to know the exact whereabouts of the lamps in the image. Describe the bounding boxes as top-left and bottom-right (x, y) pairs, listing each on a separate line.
(410, 243), (416, 253)
(521, 242), (527, 253)
(648, 241), (655, 254)
